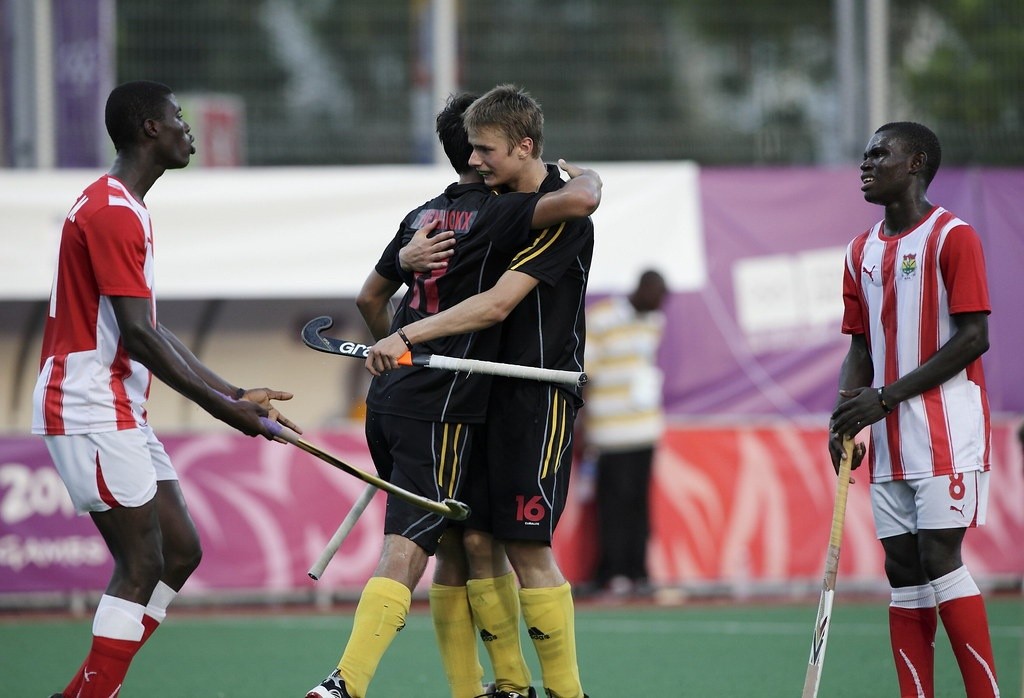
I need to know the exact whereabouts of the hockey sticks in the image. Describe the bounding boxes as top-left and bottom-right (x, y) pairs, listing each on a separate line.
(308, 474), (383, 580)
(801, 433), (855, 698)
(210, 387), (471, 521)
(300, 316), (587, 388)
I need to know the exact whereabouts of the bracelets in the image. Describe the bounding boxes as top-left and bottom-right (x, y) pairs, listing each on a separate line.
(397, 328), (414, 352)
(876, 387), (894, 414)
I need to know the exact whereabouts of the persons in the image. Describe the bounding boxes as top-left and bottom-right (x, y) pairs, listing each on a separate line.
(306, 95), (604, 698)
(367, 85), (590, 696)
(32, 79), (304, 698)
(581, 269), (672, 597)
(828, 121), (999, 696)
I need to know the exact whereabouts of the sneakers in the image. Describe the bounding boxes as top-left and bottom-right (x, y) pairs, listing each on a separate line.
(485, 682), (538, 698)
(305, 667), (353, 698)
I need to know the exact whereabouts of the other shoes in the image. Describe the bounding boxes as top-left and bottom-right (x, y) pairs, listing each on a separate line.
(607, 574), (653, 598)
